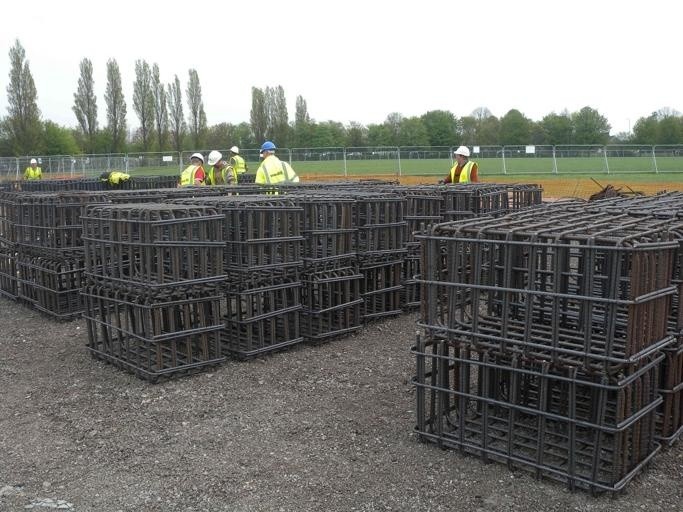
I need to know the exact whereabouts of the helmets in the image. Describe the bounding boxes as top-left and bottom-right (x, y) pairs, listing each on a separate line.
(230, 146), (239, 153)
(31, 159), (37, 164)
(260, 141), (276, 153)
(190, 151), (222, 166)
(454, 145), (470, 156)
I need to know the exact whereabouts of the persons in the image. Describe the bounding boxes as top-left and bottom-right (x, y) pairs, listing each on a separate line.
(22, 158), (41, 181)
(201, 150), (239, 196)
(228, 146), (248, 175)
(175, 153), (206, 190)
(254, 141), (299, 195)
(437, 146), (478, 186)
